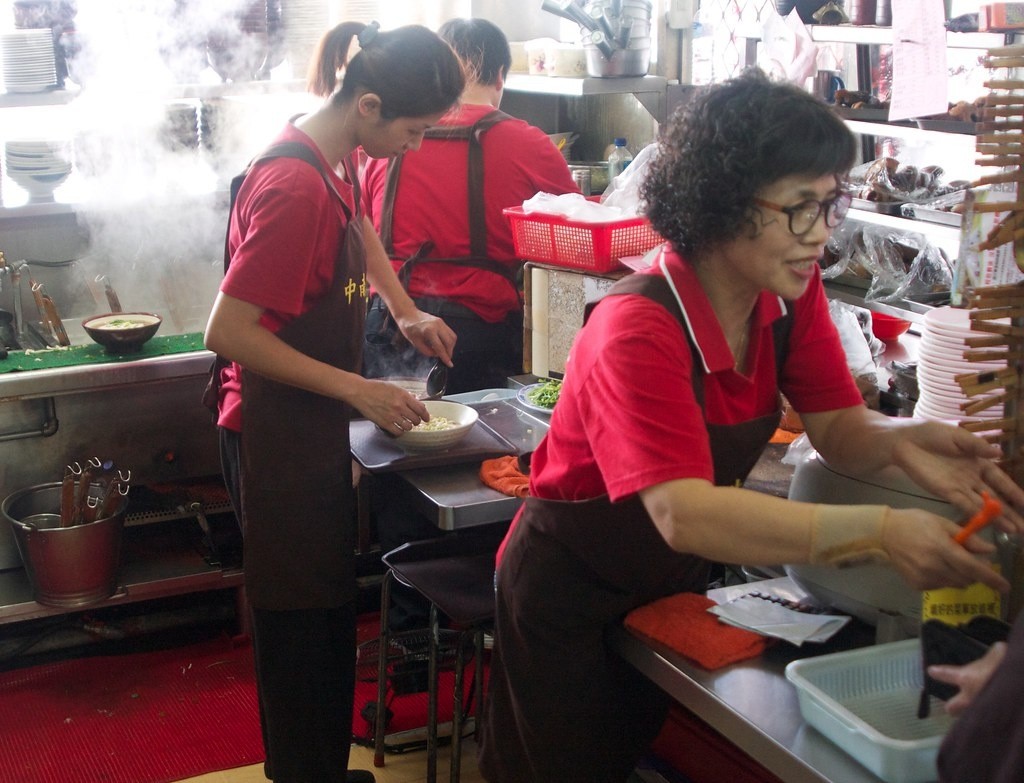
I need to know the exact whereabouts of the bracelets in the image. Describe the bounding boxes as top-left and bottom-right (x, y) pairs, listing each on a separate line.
(808, 500), (892, 573)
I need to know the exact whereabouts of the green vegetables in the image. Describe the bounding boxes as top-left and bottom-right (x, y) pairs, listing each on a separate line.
(525, 378), (562, 407)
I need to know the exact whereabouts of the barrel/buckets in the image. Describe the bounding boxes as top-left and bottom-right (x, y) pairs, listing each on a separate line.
(1, 482), (128, 608)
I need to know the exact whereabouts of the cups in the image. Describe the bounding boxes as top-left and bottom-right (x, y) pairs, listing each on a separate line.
(818, 69), (844, 103)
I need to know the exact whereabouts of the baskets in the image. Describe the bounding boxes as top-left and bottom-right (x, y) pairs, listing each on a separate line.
(503, 196), (668, 274)
(784, 639), (948, 781)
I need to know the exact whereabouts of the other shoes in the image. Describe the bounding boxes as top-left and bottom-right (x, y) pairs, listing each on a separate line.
(344, 770), (375, 783)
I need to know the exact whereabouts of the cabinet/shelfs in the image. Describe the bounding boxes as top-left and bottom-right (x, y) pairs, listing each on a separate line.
(672, 0), (1024, 401)
(0, 79), (86, 220)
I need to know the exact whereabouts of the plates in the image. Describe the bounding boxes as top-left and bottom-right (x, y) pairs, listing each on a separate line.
(911, 307), (1007, 457)
(517, 383), (554, 414)
(0, 29), (58, 94)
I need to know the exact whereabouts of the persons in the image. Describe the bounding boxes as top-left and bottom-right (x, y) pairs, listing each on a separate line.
(920, 614), (1024, 783)
(495, 67), (1024, 783)
(197, 21), (462, 783)
(352, 16), (583, 693)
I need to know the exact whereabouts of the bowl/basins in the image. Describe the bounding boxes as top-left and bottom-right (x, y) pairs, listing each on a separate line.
(369, 376), (445, 400)
(546, 48), (586, 78)
(6, 141), (72, 198)
(566, 161), (610, 194)
(528, 50), (546, 75)
(509, 43), (527, 72)
(375, 400), (479, 457)
(872, 313), (911, 341)
(82, 311), (163, 353)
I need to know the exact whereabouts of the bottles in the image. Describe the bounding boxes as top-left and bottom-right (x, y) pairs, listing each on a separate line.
(609, 138), (632, 186)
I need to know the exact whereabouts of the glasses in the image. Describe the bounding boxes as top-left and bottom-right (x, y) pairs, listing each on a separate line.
(749, 191), (853, 236)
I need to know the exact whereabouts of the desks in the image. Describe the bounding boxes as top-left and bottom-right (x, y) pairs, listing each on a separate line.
(618, 630), (883, 783)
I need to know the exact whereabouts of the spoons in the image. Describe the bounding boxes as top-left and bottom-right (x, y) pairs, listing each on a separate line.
(427, 358), (447, 395)
